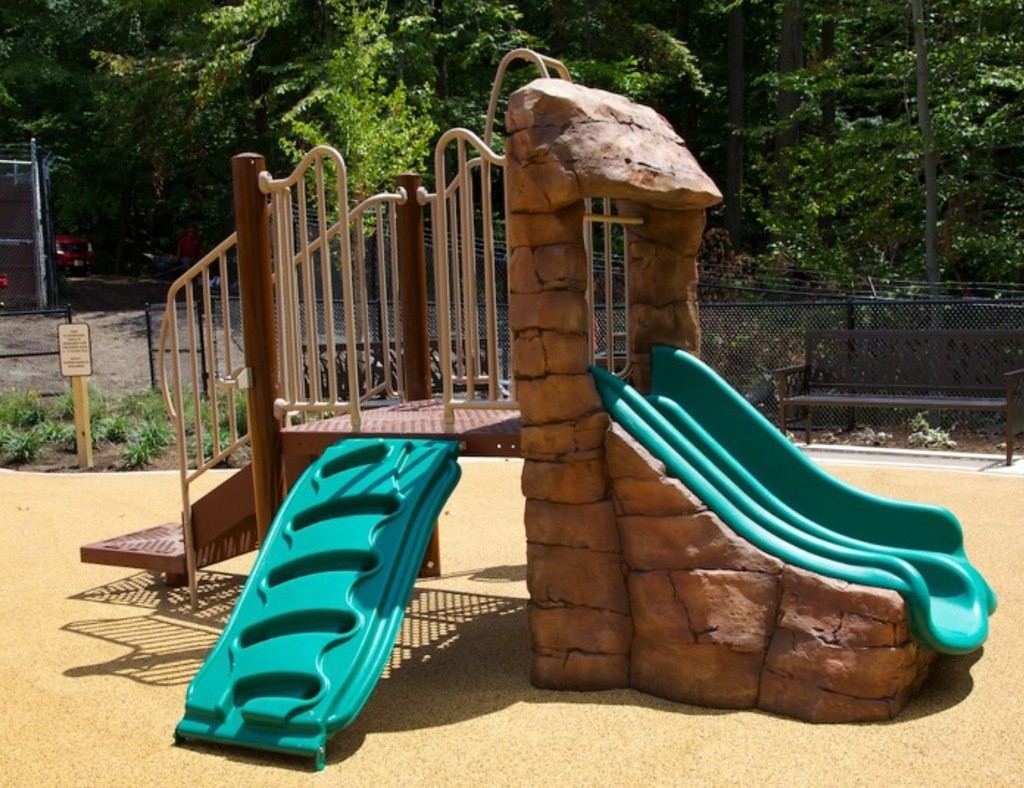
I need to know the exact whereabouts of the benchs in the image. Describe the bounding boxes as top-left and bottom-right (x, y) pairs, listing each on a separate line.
(776, 326), (1024, 466)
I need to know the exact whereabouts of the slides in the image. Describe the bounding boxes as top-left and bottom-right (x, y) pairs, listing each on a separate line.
(587, 346), (1000, 656)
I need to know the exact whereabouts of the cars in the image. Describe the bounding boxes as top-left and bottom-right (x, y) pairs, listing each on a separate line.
(51, 224), (96, 277)
